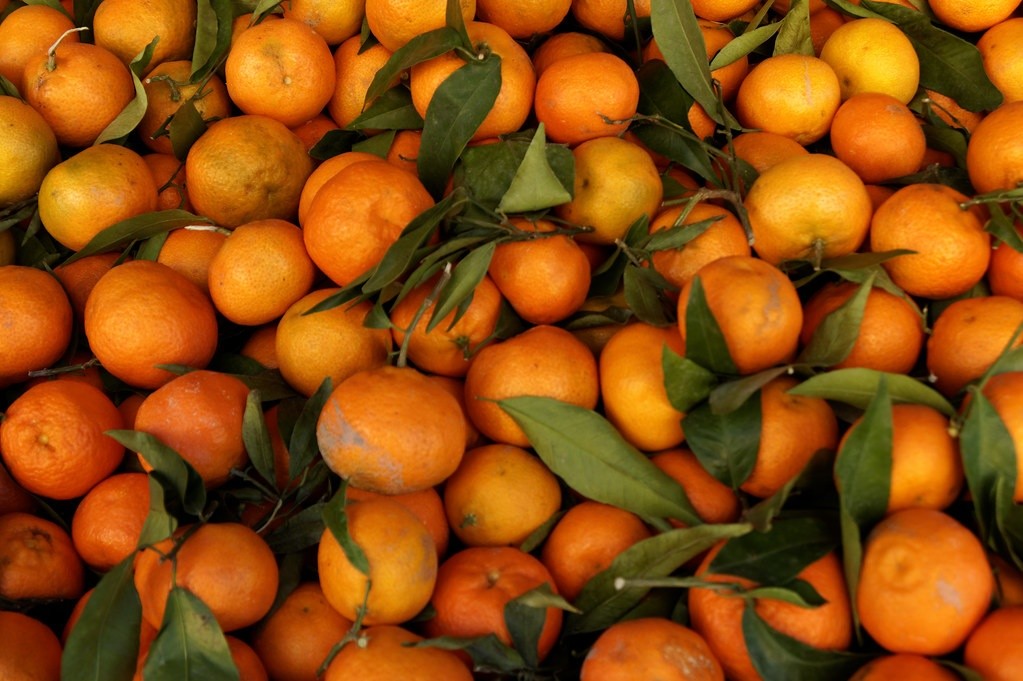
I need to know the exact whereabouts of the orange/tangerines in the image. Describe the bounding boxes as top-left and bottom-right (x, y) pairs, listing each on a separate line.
(0, 0), (1023, 681)
